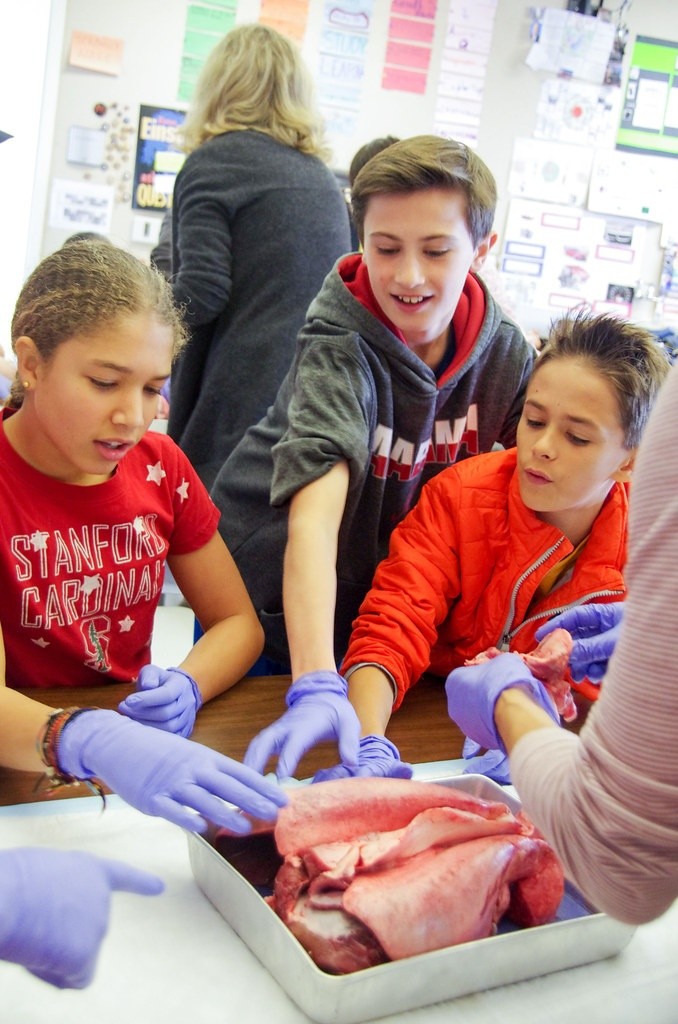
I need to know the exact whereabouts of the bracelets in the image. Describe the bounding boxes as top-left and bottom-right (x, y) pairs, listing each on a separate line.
(37, 702), (111, 811)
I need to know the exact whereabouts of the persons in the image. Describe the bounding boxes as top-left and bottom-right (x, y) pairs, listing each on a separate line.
(213, 135), (542, 787)
(167, 22), (354, 676)
(307, 309), (673, 785)
(445, 353), (678, 925)
(0, 844), (166, 990)
(1, 230), (295, 837)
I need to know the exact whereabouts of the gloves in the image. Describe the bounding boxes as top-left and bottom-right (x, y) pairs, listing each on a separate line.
(445, 652), (562, 785)
(535, 602), (625, 683)
(0, 847), (165, 990)
(311, 735), (414, 784)
(118, 664), (202, 739)
(242, 671), (361, 777)
(58, 707), (286, 834)
